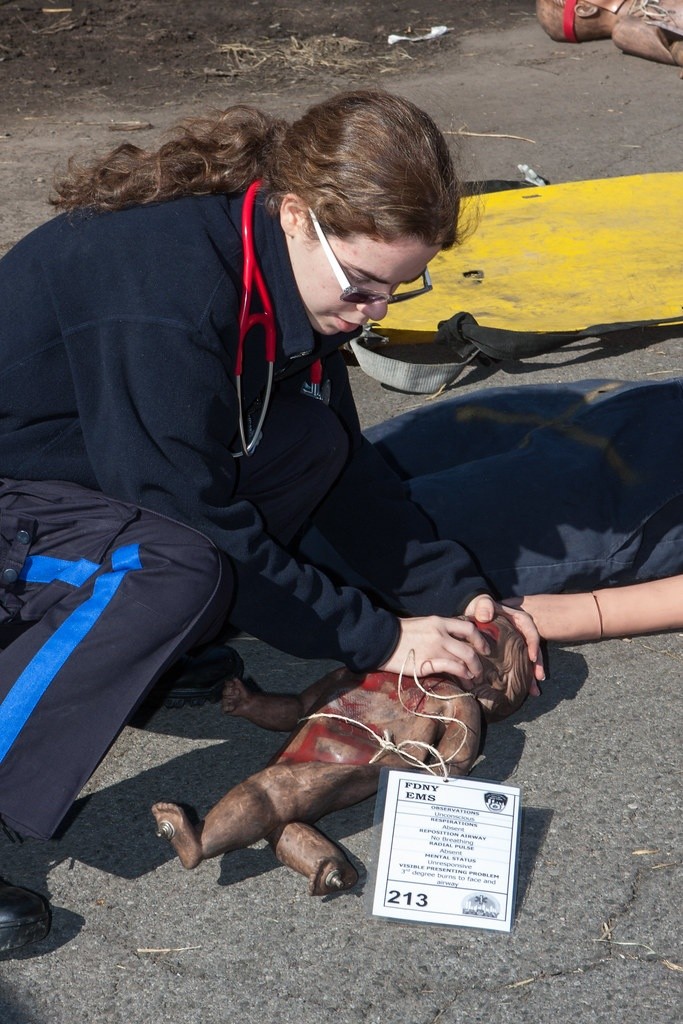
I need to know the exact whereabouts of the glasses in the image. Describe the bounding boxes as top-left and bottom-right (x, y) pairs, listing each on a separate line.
(306, 205), (432, 306)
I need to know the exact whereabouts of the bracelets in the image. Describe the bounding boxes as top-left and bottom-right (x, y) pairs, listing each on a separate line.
(591, 591), (603, 640)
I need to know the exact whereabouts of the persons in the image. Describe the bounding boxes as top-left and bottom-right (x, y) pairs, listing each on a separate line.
(361, 377), (683, 639)
(152, 612), (533, 898)
(536, 0), (683, 66)
(0, 91), (543, 965)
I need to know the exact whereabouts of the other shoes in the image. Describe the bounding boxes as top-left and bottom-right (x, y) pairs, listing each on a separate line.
(0, 874), (52, 953)
(150, 642), (246, 709)
